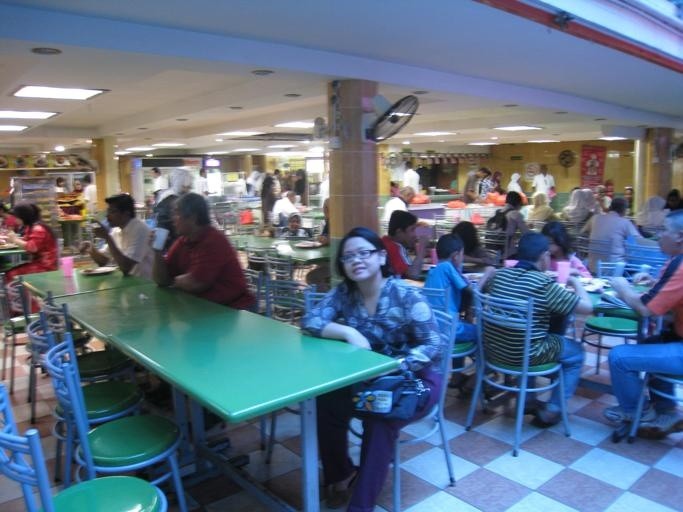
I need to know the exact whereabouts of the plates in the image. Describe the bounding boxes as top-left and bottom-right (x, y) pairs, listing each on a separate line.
(79, 266), (119, 275)
(294, 241), (323, 249)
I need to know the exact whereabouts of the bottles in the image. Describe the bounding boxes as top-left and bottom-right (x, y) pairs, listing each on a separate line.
(95, 220), (109, 249)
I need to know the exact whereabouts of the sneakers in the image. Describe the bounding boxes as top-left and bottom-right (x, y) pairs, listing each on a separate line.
(604, 403), (658, 422)
(536, 402), (563, 425)
(327, 470), (359, 509)
(638, 409), (683, 439)
(524, 398), (545, 414)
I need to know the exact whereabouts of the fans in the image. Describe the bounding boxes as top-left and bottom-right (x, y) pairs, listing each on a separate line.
(361, 93), (418, 143)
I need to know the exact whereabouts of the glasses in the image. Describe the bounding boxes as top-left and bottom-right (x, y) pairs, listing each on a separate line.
(338, 247), (384, 263)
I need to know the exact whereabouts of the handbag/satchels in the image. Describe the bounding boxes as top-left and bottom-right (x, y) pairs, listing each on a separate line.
(350, 371), (430, 422)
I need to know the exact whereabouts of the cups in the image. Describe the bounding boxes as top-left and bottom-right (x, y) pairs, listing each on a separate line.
(61, 258), (75, 276)
(556, 262), (571, 284)
(431, 248), (437, 264)
(152, 227), (171, 251)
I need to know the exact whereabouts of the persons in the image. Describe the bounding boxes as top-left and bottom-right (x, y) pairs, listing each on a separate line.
(379, 163), (683, 439)
(245, 160), (337, 291)
(1, 202), (57, 331)
(146, 193), (256, 441)
(55, 172), (98, 251)
(299, 227), (443, 512)
(142, 196), (179, 411)
(78, 194), (156, 283)
(146, 168), (168, 194)
(195, 169), (209, 198)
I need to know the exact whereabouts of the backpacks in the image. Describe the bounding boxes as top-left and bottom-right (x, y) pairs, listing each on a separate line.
(485, 209), (516, 250)
